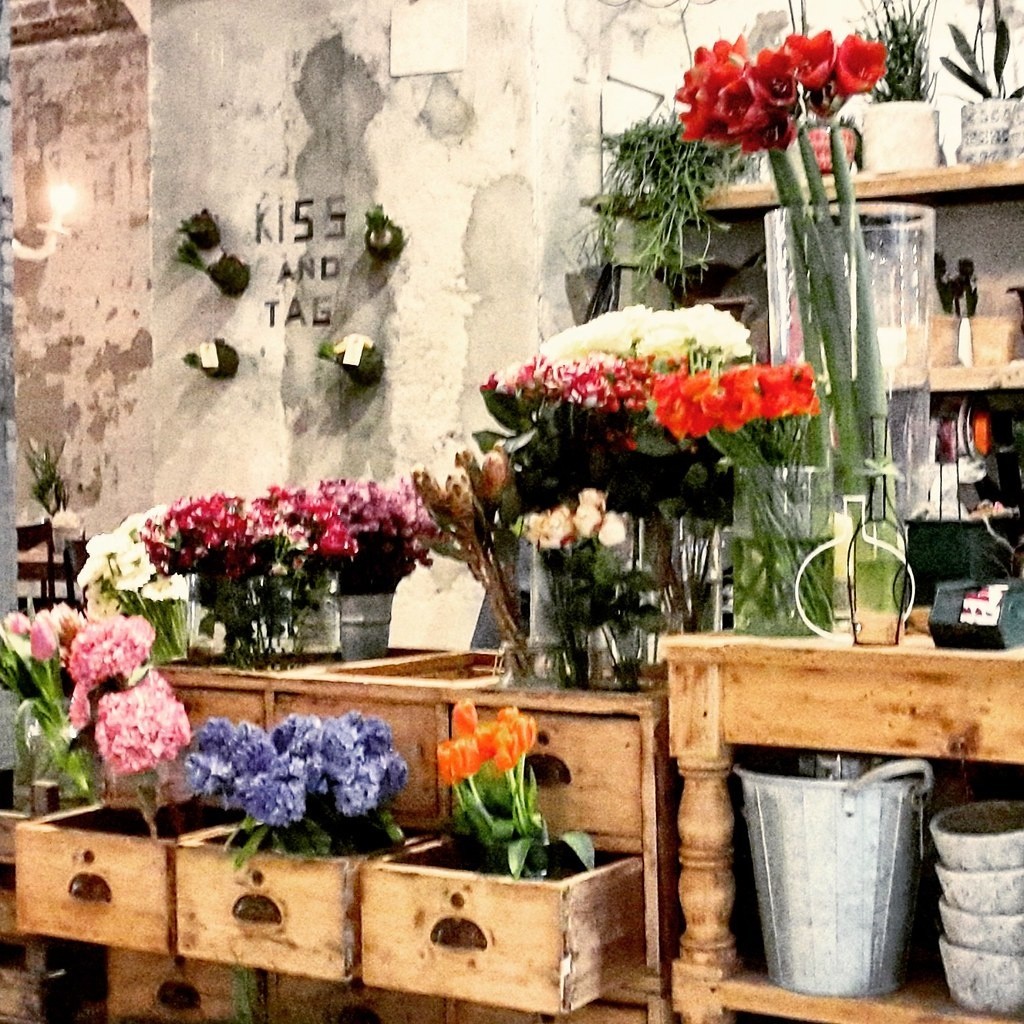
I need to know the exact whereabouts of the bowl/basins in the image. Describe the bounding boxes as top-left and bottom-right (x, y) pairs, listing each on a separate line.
(930, 801), (1024, 1014)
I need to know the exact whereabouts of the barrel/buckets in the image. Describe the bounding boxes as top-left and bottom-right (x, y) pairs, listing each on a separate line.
(735, 759), (933, 999)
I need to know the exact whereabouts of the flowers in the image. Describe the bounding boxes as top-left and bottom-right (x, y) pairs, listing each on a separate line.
(648, 363), (835, 625)
(308, 476), (434, 592)
(184, 710), (404, 865)
(436, 698), (596, 880)
(471, 302), (754, 689)
(137, 482), (359, 668)
(677, 28), (905, 608)
(0, 601), (194, 839)
(77, 502), (199, 668)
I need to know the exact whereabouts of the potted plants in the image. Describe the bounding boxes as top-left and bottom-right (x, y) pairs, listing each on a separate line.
(857, 0), (942, 172)
(802, 116), (857, 175)
(938, 0), (1024, 165)
(24, 438), (80, 552)
(928, 799), (1024, 871)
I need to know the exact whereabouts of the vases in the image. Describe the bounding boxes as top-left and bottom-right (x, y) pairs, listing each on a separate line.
(142, 598), (187, 664)
(758, 200), (937, 626)
(638, 513), (724, 633)
(543, 554), (649, 690)
(230, 575), (291, 670)
(731, 463), (837, 637)
(930, 861), (1022, 1016)
(336, 592), (394, 661)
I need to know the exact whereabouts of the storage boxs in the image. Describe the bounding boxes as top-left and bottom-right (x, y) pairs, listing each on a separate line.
(904, 508), (1018, 606)
(0, 779), (104, 1024)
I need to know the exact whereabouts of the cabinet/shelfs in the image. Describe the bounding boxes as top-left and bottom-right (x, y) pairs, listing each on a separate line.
(14, 648), (684, 1024)
(658, 606), (1024, 1024)
(581, 157), (1024, 393)
